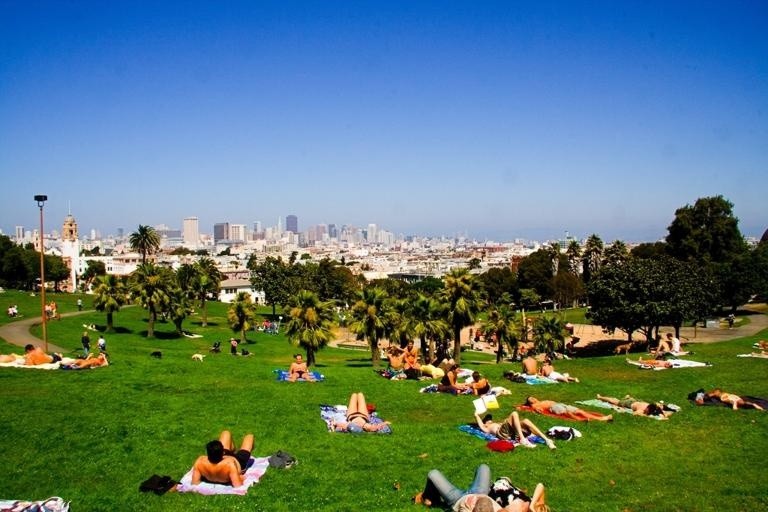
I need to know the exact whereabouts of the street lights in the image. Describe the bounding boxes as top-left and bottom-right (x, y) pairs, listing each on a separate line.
(33, 193), (49, 355)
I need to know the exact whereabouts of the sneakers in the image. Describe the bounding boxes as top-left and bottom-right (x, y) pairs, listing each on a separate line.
(545, 439), (557, 450)
(520, 438), (536, 449)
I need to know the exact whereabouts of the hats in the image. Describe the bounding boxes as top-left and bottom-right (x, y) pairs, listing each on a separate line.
(482, 414), (492, 424)
